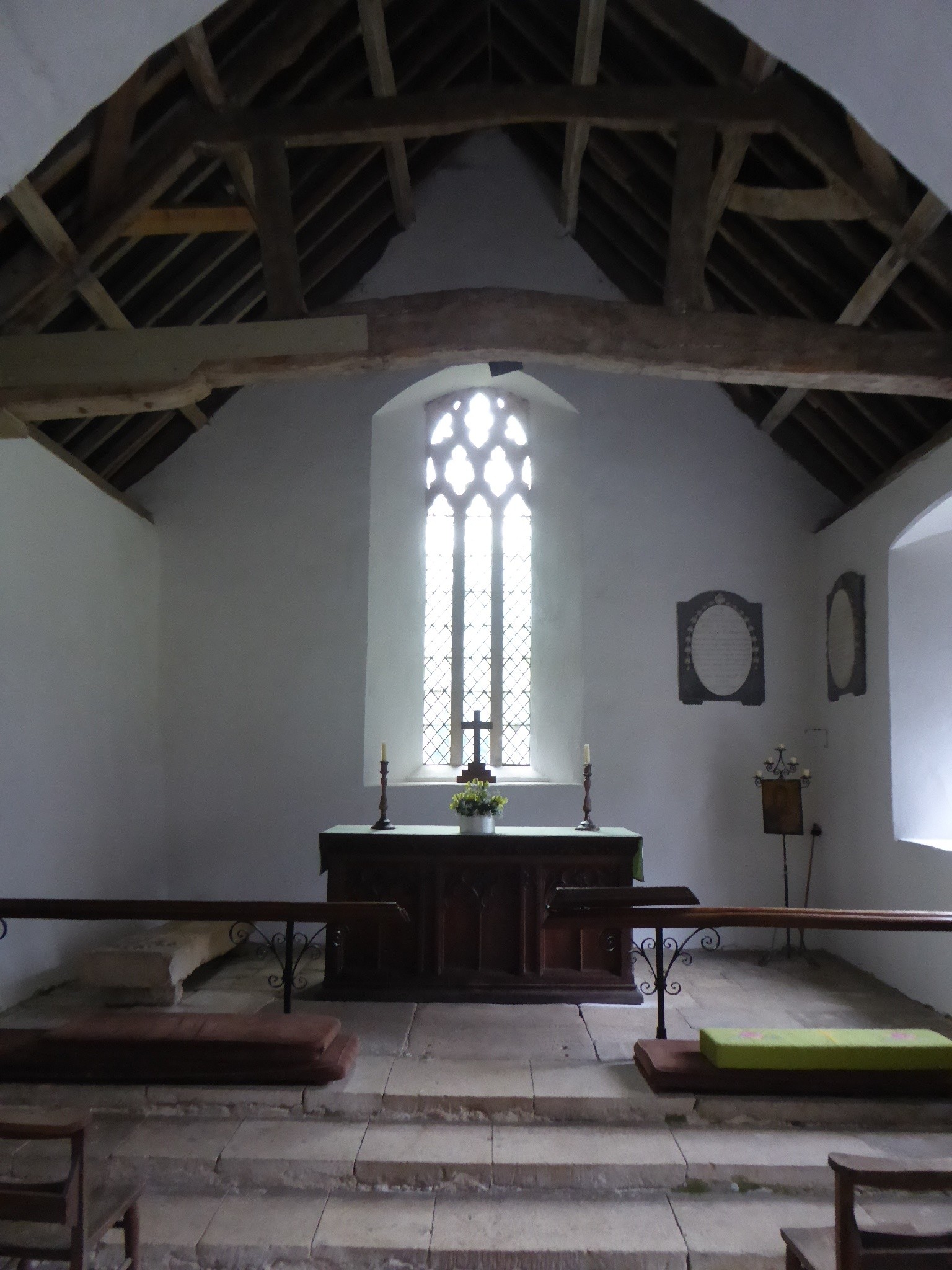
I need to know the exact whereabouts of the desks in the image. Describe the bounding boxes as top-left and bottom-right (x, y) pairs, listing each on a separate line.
(318, 825), (645, 1004)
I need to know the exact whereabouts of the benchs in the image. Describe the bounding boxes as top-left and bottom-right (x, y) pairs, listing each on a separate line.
(81, 920), (257, 1006)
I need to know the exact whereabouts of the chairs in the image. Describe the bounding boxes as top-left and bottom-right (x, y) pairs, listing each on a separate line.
(0, 1106), (146, 1270)
(780, 1152), (952, 1270)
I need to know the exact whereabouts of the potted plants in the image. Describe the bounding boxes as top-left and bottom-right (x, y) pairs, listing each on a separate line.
(450, 778), (508, 833)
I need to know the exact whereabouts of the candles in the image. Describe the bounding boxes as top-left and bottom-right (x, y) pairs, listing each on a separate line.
(778, 744), (784, 749)
(382, 743), (387, 761)
(791, 756), (796, 764)
(584, 744), (590, 764)
(756, 770), (762, 777)
(766, 757), (773, 763)
(804, 769), (809, 777)
